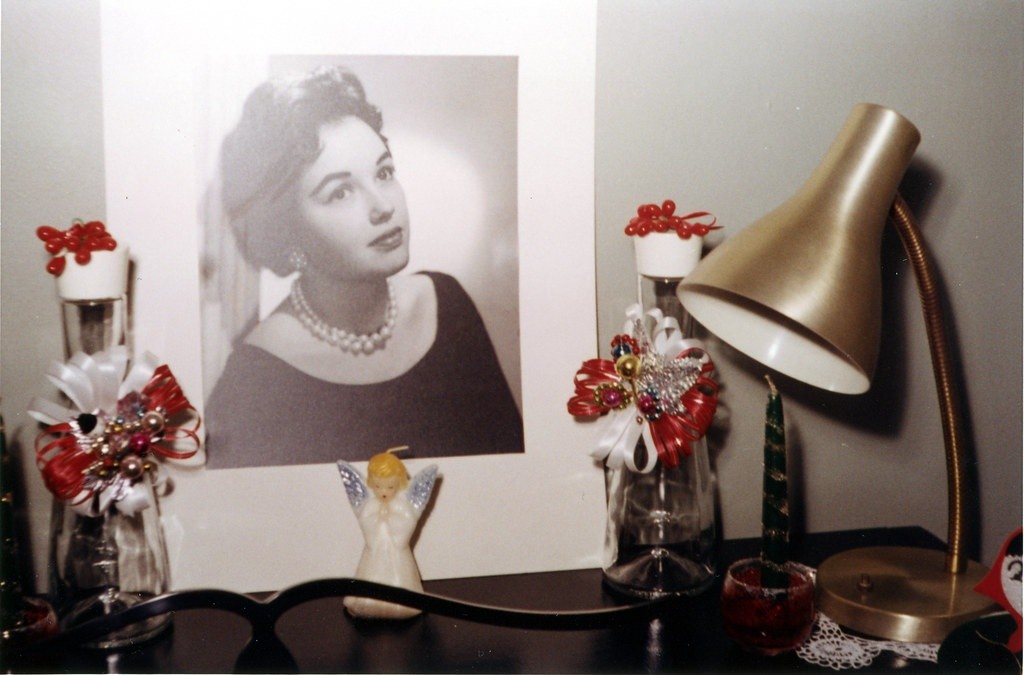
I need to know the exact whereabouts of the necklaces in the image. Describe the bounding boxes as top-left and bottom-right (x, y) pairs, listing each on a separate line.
(290, 278), (397, 355)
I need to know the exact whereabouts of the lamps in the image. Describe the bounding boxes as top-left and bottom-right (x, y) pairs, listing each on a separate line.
(674, 105), (999, 644)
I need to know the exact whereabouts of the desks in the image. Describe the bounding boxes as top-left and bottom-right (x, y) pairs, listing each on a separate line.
(1, 524), (1022, 675)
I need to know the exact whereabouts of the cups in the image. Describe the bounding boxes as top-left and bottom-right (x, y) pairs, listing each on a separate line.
(0, 594), (55, 674)
(720, 556), (816, 658)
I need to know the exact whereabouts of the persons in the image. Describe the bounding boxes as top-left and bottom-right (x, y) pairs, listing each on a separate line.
(975, 527), (1022, 651)
(206, 65), (522, 469)
(338, 453), (440, 616)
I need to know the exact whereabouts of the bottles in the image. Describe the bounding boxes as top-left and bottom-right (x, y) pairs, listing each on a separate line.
(602, 229), (723, 599)
(45, 250), (177, 649)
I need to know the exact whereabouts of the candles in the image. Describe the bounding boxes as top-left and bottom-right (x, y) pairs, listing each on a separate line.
(762, 373), (791, 593)
